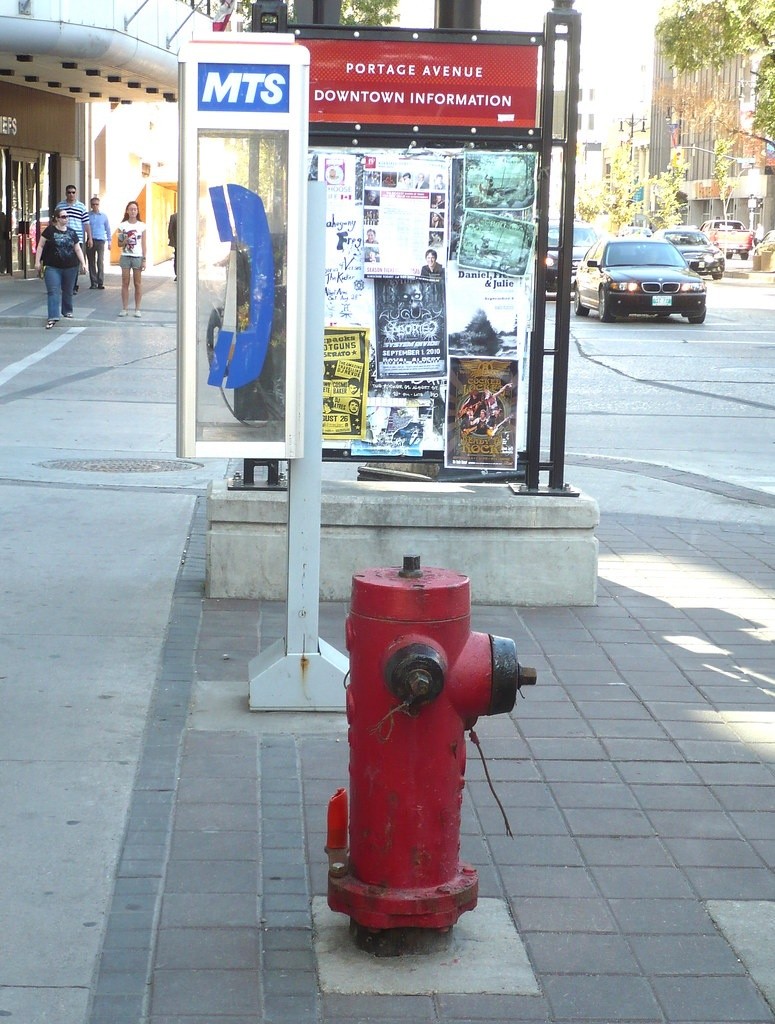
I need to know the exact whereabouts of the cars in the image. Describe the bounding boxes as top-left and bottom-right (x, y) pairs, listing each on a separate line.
(653, 227), (725, 281)
(573, 236), (707, 325)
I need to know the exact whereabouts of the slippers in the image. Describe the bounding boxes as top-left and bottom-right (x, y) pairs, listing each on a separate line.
(46, 321), (55, 329)
(62, 312), (73, 318)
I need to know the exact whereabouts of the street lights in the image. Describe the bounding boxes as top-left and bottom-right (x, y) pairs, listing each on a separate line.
(618, 114), (649, 194)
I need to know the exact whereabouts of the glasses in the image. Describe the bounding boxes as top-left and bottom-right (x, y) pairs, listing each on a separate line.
(66, 192), (76, 194)
(91, 204), (98, 206)
(58, 215), (69, 219)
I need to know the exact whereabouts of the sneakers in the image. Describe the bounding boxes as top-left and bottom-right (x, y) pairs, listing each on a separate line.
(118, 310), (128, 317)
(89, 284), (97, 289)
(98, 284), (105, 289)
(134, 310), (141, 318)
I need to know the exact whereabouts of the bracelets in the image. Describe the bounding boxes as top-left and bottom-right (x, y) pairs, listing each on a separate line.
(142, 257), (146, 262)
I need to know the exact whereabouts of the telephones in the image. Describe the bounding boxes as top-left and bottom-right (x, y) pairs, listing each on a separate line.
(204, 183), (278, 430)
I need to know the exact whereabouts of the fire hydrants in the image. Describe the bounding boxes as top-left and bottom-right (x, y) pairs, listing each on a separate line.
(319, 554), (537, 963)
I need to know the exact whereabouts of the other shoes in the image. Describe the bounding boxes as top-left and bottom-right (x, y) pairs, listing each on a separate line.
(174, 276), (177, 281)
(73, 289), (77, 295)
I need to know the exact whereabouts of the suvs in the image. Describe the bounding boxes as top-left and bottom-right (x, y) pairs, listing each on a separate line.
(545, 218), (603, 296)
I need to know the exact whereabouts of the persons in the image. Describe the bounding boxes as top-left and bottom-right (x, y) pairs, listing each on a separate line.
(117, 202), (146, 317)
(54, 185), (93, 295)
(82, 197), (112, 290)
(309, 151), (523, 442)
(34, 209), (87, 329)
(168, 213), (178, 281)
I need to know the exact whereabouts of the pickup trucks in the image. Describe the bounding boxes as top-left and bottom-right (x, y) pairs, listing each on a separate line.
(700, 220), (753, 260)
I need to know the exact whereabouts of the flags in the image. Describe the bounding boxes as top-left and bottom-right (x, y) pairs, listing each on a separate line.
(212, 0), (235, 31)
